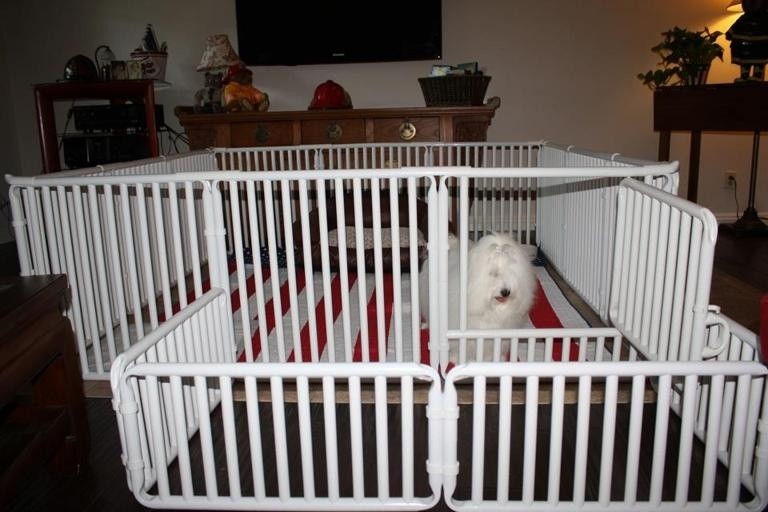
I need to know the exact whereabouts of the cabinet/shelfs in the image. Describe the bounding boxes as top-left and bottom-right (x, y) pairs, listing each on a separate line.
(29, 75), (173, 180)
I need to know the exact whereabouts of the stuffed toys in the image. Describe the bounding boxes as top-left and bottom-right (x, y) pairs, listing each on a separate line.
(221, 64), (270, 113)
(193, 71), (225, 113)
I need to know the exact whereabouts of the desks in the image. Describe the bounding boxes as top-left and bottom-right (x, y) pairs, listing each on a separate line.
(166, 95), (503, 226)
(0, 268), (98, 512)
(652, 80), (767, 203)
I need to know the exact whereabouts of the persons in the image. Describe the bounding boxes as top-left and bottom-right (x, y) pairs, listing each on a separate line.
(112, 62), (142, 81)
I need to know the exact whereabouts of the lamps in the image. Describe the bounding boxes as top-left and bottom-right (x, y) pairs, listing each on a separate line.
(196, 31), (246, 113)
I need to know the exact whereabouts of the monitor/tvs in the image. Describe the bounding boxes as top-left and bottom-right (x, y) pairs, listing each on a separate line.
(235, 0), (442, 66)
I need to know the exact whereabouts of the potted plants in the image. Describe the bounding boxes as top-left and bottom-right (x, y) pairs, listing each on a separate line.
(638, 21), (726, 95)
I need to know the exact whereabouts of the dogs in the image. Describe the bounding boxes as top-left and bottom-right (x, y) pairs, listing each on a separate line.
(418, 227), (538, 365)
